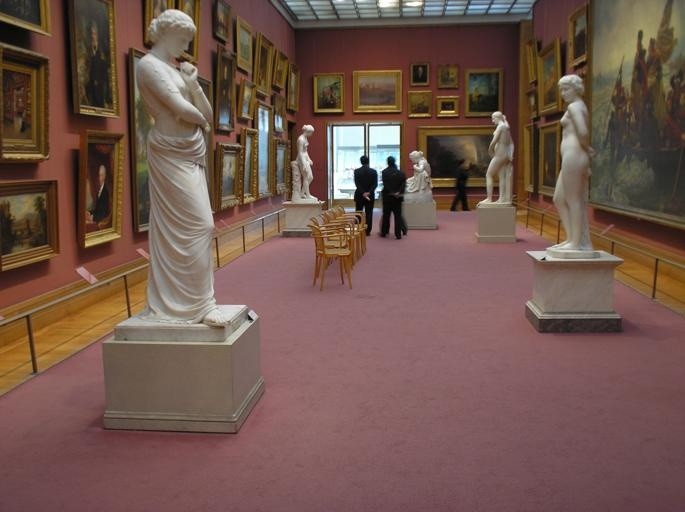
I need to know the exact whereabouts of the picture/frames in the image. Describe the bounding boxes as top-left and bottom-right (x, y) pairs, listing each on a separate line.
(67, 0), (121, 119)
(465, 67), (505, 118)
(0, 42), (50, 164)
(143, 1), (171, 47)
(0, 177), (59, 272)
(128, 46), (218, 235)
(0, 0), (52, 38)
(407, 91), (432, 119)
(522, 1), (685, 231)
(416, 125), (500, 189)
(213, 0), (301, 211)
(353, 70), (403, 113)
(437, 64), (455, 86)
(409, 64), (430, 86)
(171, 0), (201, 66)
(435, 95), (460, 118)
(78, 129), (125, 250)
(313, 72), (346, 115)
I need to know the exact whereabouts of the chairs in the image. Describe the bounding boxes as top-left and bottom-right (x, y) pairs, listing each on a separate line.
(306, 205), (368, 291)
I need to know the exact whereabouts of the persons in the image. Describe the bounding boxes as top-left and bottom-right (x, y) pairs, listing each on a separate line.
(405, 150), (433, 192)
(136, 7), (232, 327)
(84, 163), (113, 224)
(380, 166), (404, 240)
(551, 74), (599, 250)
(295, 124), (320, 203)
(479, 110), (514, 204)
(414, 65), (429, 83)
(449, 159), (472, 212)
(599, 28), (685, 156)
(351, 153), (378, 236)
(381, 155), (409, 235)
(82, 17), (110, 107)
